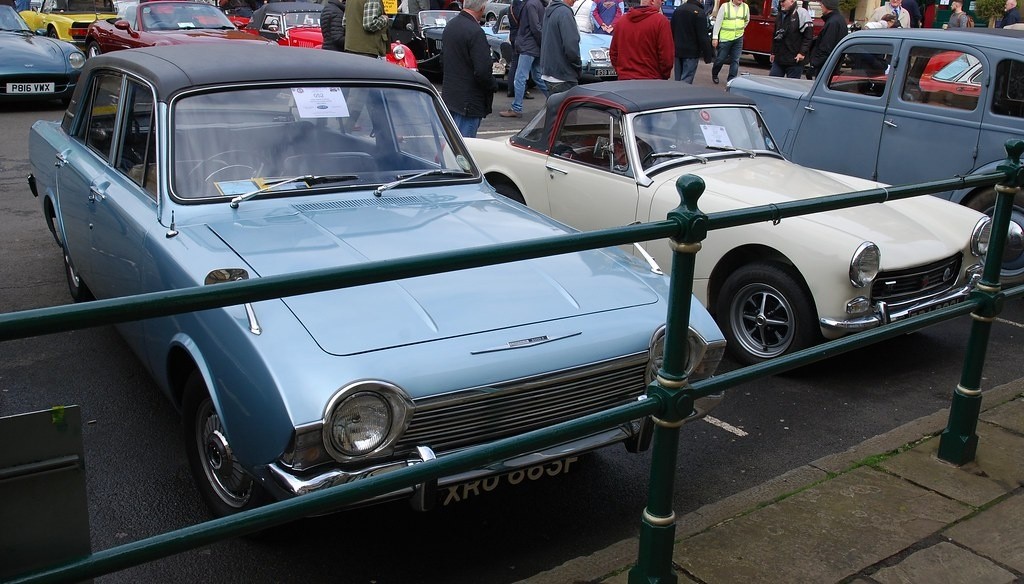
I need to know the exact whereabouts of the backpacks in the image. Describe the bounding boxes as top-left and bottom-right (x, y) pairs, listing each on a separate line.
(958, 12), (974, 28)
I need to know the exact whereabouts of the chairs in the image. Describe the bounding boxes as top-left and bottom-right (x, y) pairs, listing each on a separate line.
(284, 152), (378, 175)
(129, 159), (235, 197)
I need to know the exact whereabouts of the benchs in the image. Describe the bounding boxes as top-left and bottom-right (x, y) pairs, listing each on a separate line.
(97, 121), (321, 180)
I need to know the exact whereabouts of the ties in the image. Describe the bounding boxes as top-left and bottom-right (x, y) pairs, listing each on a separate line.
(894, 9), (898, 20)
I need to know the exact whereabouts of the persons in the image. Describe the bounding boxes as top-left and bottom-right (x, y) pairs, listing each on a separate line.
(540, 0), (582, 124)
(712, 0), (751, 83)
(806, 0), (848, 80)
(397, 0), (464, 15)
(441, 0), (498, 138)
(999, 0), (1021, 28)
(337, 0), (404, 142)
(670, 0), (711, 84)
(609, 0), (675, 80)
(499, 0), (550, 118)
(573, 0), (624, 37)
(868, 0), (921, 28)
(845, 20), (888, 75)
(768, 0), (813, 79)
(947, 0), (968, 28)
(316, 0), (362, 131)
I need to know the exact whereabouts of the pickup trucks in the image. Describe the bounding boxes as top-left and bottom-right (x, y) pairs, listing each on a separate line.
(726, 27), (1023, 305)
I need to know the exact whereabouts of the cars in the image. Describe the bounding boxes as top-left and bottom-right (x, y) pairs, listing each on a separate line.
(23, 43), (728, 541)
(414, 0), (1024, 94)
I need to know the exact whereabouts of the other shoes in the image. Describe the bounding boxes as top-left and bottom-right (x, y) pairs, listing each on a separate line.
(712, 74), (719, 84)
(499, 109), (522, 117)
(524, 91), (534, 99)
(507, 93), (515, 98)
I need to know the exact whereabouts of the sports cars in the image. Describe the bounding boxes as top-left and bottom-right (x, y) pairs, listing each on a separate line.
(17, 0), (123, 52)
(432, 77), (995, 376)
(85, 0), (281, 70)
(0, 0), (89, 109)
(220, 0), (420, 74)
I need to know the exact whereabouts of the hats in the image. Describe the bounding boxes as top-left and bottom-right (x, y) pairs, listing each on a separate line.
(822, 0), (838, 10)
